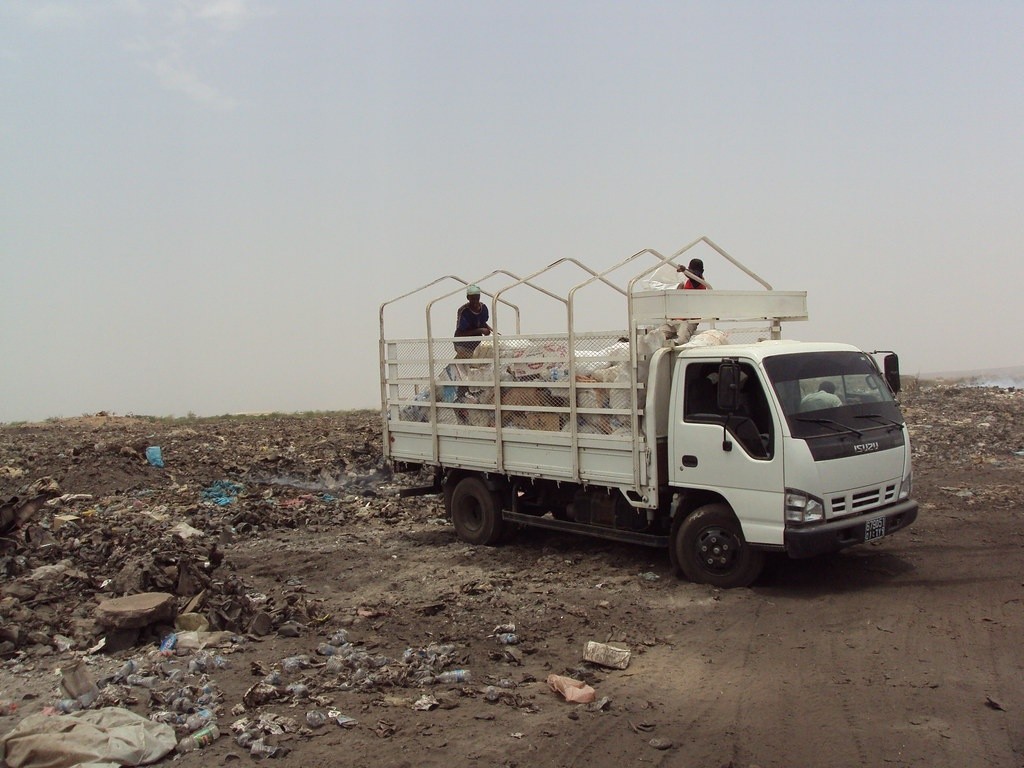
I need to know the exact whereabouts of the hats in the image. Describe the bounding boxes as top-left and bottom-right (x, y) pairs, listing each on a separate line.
(467, 284), (481, 295)
(688, 259), (704, 271)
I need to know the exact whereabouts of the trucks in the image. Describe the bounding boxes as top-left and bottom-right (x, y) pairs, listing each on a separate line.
(378, 237), (920, 586)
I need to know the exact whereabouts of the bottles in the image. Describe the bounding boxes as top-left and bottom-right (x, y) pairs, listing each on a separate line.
(45, 699), (80, 713)
(316, 642), (342, 656)
(550, 367), (559, 380)
(172, 725), (219, 753)
(122, 675), (158, 687)
(183, 708), (215, 731)
(434, 669), (471, 682)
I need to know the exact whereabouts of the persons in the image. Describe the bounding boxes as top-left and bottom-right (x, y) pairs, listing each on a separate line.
(453, 285), (491, 359)
(719, 369), (770, 439)
(799, 381), (842, 412)
(617, 259), (706, 346)
